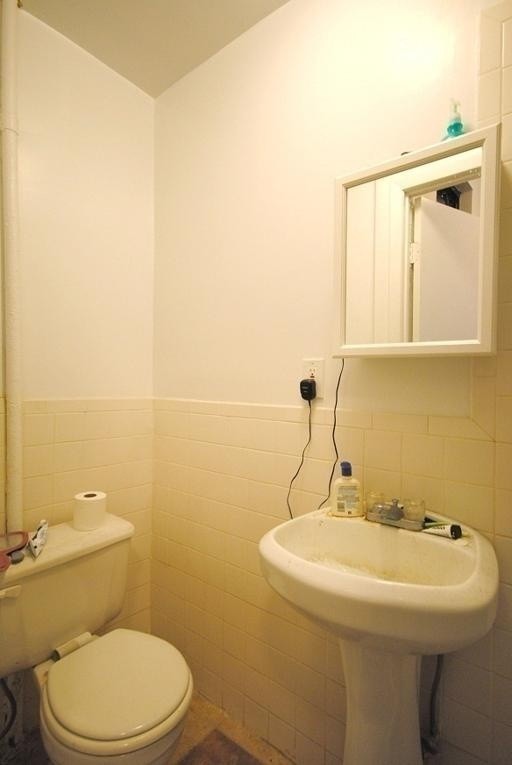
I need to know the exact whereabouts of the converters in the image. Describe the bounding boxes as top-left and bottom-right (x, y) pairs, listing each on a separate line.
(300, 379), (316, 400)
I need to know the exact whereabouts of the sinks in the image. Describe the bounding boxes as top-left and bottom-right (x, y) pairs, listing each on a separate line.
(255, 501), (501, 657)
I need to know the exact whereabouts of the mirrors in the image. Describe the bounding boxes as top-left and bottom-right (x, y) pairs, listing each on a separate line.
(329, 118), (507, 363)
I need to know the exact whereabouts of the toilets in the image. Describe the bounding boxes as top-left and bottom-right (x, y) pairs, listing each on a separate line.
(0, 512), (196, 765)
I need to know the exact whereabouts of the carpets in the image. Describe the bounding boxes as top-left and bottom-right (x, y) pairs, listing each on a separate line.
(174, 726), (264, 765)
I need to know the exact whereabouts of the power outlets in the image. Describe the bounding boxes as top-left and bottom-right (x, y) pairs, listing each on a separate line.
(300, 355), (325, 402)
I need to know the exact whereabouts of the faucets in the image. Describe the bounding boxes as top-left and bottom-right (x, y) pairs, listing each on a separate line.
(371, 498), (404, 522)
